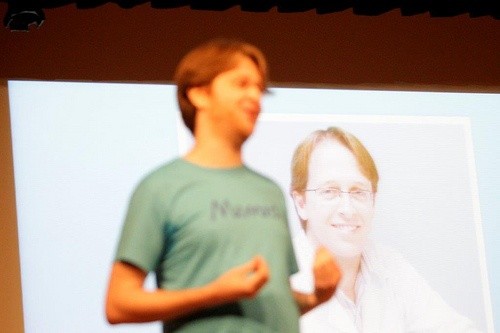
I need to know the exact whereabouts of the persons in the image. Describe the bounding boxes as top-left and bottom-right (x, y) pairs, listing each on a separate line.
(286, 121), (482, 333)
(105, 35), (343, 332)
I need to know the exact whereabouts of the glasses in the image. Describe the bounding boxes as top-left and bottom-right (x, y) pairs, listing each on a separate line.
(299, 188), (373, 205)
